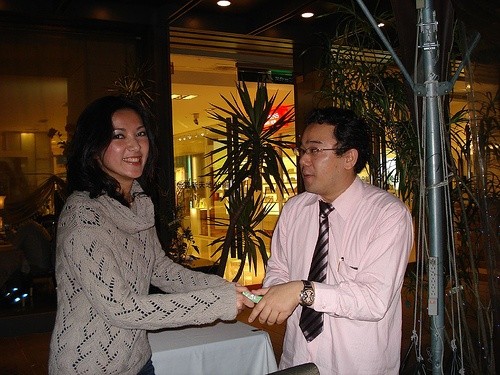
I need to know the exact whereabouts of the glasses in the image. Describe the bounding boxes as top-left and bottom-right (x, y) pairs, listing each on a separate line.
(293, 147), (348, 156)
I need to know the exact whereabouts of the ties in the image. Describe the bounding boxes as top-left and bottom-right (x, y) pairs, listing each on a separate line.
(299, 200), (335, 343)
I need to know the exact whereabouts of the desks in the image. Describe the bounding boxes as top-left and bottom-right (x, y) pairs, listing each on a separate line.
(0, 250), (25, 290)
(147, 320), (268, 375)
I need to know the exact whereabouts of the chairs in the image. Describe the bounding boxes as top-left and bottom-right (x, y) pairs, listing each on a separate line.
(22, 240), (57, 309)
